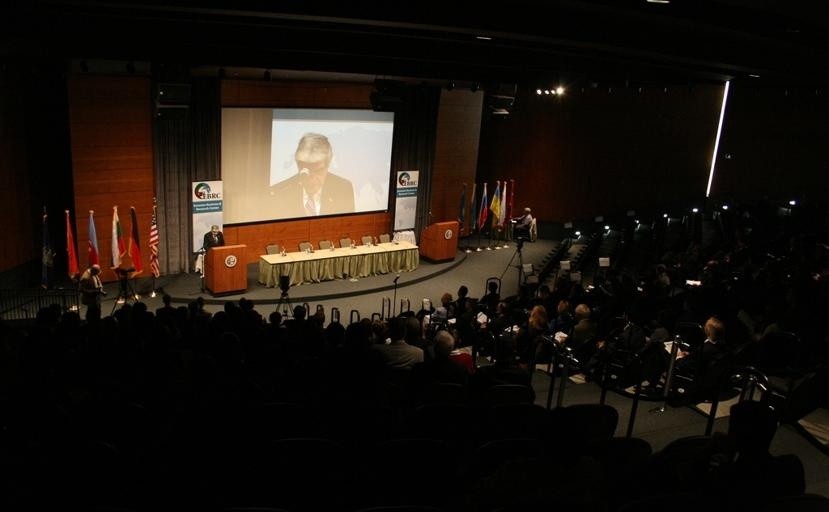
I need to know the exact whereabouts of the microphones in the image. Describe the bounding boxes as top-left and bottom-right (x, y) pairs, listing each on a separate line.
(298, 168), (309, 186)
(393, 274), (400, 283)
(429, 211), (434, 219)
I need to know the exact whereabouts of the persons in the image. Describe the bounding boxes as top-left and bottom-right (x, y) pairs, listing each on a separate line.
(511, 208), (534, 240)
(270, 133), (354, 216)
(79, 264), (107, 321)
(2, 295), (829, 510)
(454, 208), (829, 360)
(204, 226), (225, 253)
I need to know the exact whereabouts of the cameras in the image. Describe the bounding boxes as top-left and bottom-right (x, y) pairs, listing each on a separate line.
(98, 286), (107, 296)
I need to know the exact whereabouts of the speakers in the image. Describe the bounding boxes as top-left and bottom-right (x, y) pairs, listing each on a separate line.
(400, 87), (432, 128)
(192, 77), (216, 112)
(485, 94), (516, 120)
(152, 83), (192, 109)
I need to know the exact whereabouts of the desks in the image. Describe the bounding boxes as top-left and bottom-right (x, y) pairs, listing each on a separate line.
(260, 242), (419, 288)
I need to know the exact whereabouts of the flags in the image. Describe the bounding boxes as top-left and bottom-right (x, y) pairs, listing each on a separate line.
(128, 209), (145, 273)
(40, 215), (54, 292)
(109, 210), (127, 270)
(456, 179), (515, 237)
(149, 211), (162, 279)
(65, 213), (80, 280)
(88, 214), (99, 266)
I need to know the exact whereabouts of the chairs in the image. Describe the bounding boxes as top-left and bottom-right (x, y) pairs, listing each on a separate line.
(264, 234), (390, 255)
(518, 214), (829, 458)
(4, 310), (828, 511)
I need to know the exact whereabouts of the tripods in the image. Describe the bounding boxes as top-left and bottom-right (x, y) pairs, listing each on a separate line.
(499, 247), (523, 285)
(189, 242), (211, 296)
(111, 275), (138, 315)
(275, 293), (294, 319)
